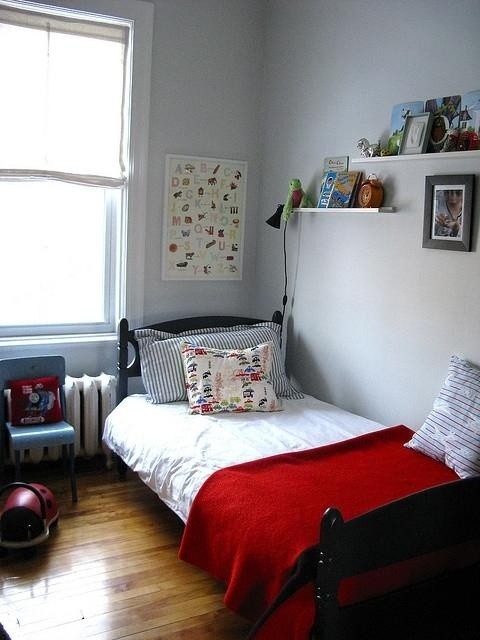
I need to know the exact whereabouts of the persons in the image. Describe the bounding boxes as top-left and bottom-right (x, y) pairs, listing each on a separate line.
(434, 190), (464, 239)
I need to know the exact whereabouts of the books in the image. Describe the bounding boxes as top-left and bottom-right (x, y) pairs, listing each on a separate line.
(316, 156), (363, 208)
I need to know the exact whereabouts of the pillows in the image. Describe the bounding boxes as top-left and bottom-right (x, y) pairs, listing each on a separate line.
(402, 355), (480, 482)
(7, 376), (63, 427)
(134, 322), (304, 416)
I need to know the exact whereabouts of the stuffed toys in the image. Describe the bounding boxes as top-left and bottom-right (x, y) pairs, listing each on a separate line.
(282, 178), (314, 223)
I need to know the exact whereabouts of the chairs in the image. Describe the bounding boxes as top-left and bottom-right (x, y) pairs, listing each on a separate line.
(0, 354), (77, 503)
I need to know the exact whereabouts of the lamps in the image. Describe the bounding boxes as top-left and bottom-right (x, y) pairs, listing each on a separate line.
(264, 203), (288, 322)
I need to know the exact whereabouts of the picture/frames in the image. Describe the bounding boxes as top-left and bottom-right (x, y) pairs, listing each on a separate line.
(397, 112), (475, 253)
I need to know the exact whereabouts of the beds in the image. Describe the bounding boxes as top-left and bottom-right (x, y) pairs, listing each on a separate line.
(103, 310), (480, 640)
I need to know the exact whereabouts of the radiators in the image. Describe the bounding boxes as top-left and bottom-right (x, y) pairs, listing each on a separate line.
(3, 372), (117, 468)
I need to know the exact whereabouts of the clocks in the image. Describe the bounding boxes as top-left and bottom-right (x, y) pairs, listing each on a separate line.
(357, 174), (384, 208)
